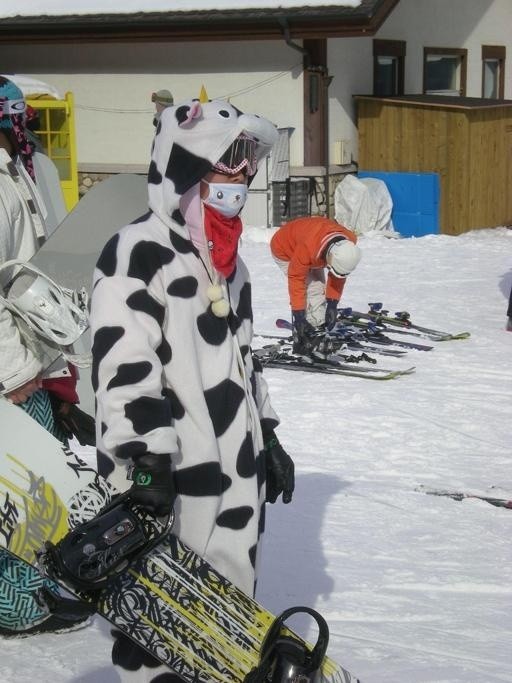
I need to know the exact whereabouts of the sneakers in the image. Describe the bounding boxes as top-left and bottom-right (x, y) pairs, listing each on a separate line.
(2, 585), (97, 639)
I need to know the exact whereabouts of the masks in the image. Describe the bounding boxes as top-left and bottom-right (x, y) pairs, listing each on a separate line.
(200, 179), (247, 219)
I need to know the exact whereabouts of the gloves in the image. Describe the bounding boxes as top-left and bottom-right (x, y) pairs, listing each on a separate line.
(130, 454), (177, 517)
(325, 301), (337, 331)
(263, 429), (295, 503)
(292, 311), (309, 336)
(51, 396), (96, 447)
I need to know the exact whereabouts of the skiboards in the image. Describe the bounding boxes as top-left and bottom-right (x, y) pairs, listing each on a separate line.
(337, 308), (471, 341)
(276, 319), (434, 353)
(252, 345), (415, 379)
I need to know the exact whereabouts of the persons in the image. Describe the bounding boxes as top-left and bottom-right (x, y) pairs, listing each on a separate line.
(0, 74), (94, 637)
(88, 96), (296, 683)
(267, 216), (362, 356)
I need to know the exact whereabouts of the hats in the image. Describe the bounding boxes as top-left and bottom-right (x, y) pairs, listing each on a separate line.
(330, 241), (359, 275)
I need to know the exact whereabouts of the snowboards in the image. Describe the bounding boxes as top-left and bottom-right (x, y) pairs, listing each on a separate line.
(0, 395), (360, 683)
(7, 173), (151, 371)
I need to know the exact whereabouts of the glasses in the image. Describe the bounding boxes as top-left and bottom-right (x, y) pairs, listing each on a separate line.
(216, 135), (259, 175)
